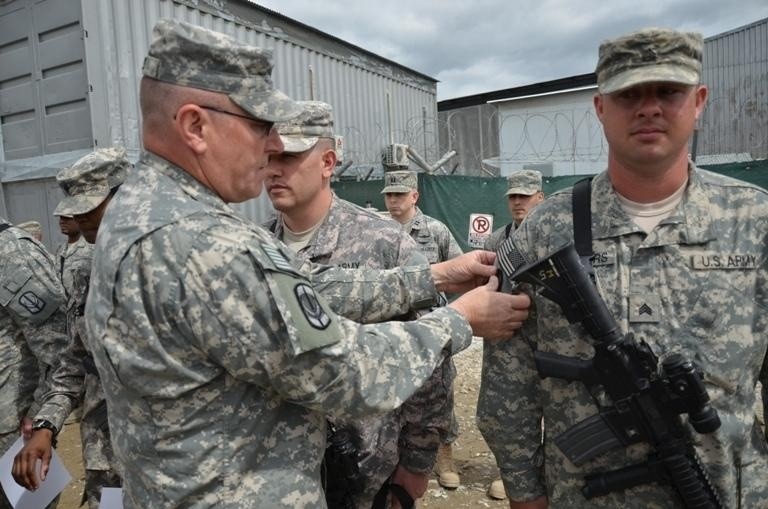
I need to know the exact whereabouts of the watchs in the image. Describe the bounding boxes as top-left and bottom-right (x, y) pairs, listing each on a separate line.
(27, 418), (58, 439)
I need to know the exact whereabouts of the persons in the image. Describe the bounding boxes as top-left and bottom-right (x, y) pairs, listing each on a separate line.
(368, 166), (467, 489)
(13, 219), (44, 244)
(83, 16), (535, 509)
(9, 140), (140, 508)
(482, 169), (545, 499)
(0, 215), (73, 508)
(474, 27), (765, 508)
(51, 212), (97, 336)
(250, 100), (458, 509)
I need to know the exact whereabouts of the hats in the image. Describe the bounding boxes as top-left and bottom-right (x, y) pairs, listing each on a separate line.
(51, 145), (131, 220)
(14, 220), (43, 237)
(379, 169), (419, 196)
(503, 168), (543, 199)
(592, 27), (705, 97)
(139, 17), (306, 124)
(265, 98), (337, 155)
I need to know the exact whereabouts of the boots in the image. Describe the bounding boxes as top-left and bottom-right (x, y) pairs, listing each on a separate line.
(433, 442), (461, 490)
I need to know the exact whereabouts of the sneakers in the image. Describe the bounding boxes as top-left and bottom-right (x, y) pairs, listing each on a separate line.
(486, 472), (506, 501)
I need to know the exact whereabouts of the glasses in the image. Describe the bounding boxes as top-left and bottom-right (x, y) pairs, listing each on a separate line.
(171, 103), (275, 138)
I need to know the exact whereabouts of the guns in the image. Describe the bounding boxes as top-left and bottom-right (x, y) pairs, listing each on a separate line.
(510, 241), (725, 509)
